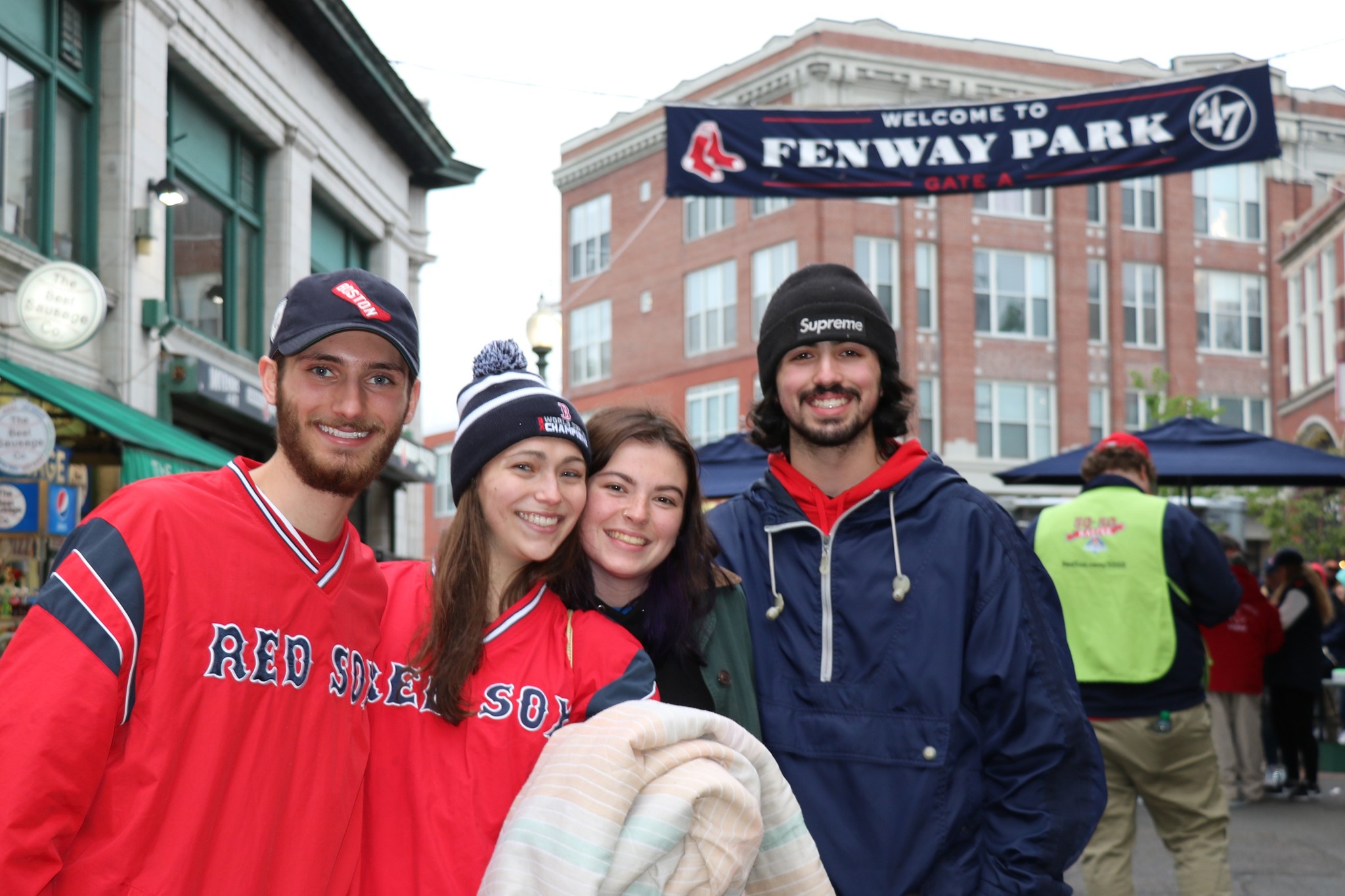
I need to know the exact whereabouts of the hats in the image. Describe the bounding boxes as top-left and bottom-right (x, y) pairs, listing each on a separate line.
(757, 264), (898, 397)
(1268, 549), (1303, 571)
(269, 268), (420, 377)
(1322, 559), (1341, 569)
(1094, 432), (1152, 464)
(450, 339), (591, 510)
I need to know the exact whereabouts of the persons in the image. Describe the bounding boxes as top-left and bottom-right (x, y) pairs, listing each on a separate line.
(1024, 431), (1243, 896)
(358, 339), (661, 896)
(703, 264), (1109, 896)
(0, 269), (421, 896)
(547, 407), (762, 743)
(1198, 535), (1345, 806)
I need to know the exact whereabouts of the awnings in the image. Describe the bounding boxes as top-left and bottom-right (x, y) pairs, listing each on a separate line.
(2, 362), (234, 492)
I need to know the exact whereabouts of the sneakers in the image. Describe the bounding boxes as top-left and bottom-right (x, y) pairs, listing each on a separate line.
(1267, 778), (1323, 800)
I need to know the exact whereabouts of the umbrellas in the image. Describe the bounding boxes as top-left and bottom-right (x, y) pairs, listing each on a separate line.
(993, 399), (1345, 511)
(693, 433), (769, 497)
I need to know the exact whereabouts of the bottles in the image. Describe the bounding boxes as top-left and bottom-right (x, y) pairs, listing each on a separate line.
(1151, 711), (1174, 733)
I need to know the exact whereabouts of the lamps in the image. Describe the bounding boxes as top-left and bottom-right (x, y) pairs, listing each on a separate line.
(135, 207), (155, 255)
(148, 178), (183, 205)
